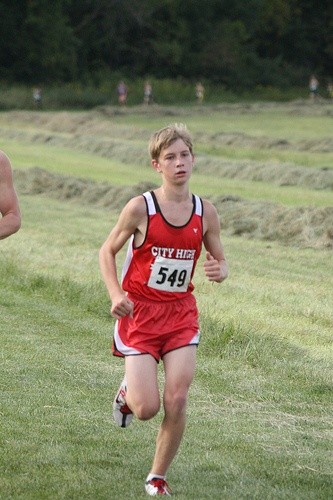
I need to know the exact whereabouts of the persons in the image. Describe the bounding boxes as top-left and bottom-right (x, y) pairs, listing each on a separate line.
(195, 82), (206, 104)
(117, 80), (129, 105)
(99, 124), (228, 497)
(143, 80), (154, 103)
(0, 151), (22, 240)
(33, 85), (43, 110)
(309, 74), (318, 99)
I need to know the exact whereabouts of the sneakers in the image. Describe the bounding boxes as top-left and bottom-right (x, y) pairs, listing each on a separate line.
(144, 477), (172, 498)
(113, 374), (134, 428)
